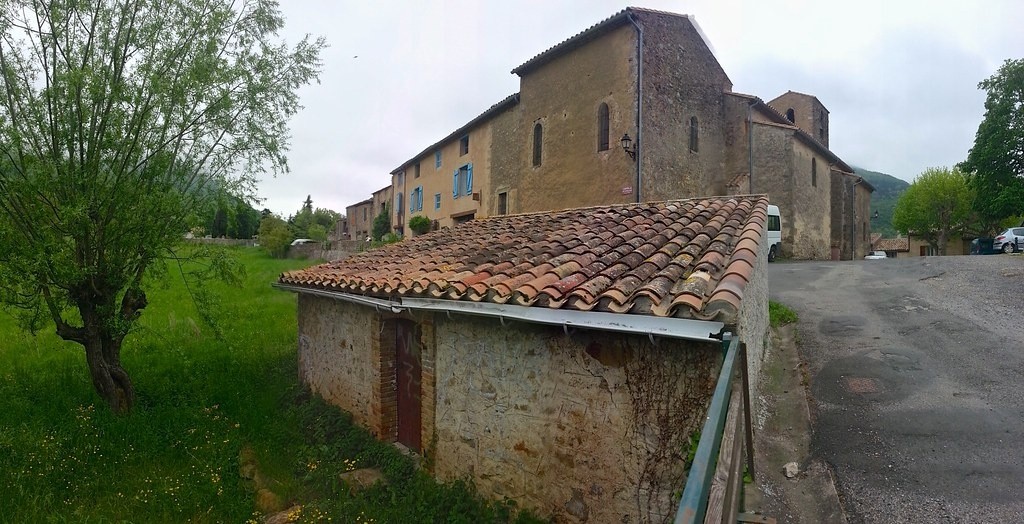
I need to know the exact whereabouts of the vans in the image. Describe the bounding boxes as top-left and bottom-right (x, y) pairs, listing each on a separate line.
(767, 205), (782, 263)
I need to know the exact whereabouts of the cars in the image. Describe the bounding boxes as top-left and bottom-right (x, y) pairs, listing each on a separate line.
(993, 227), (1024, 253)
(290, 239), (320, 248)
(864, 251), (888, 260)
(969, 236), (998, 255)
